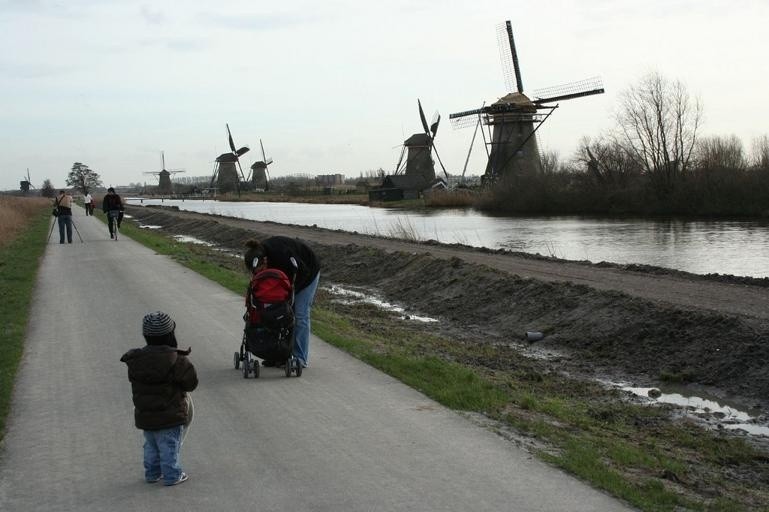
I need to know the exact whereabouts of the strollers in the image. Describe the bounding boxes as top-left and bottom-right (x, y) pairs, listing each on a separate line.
(233, 255), (306, 381)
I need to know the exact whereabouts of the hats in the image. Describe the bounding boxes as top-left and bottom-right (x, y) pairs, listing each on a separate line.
(143, 311), (177, 348)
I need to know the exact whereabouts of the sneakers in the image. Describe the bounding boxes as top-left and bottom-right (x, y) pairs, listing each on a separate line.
(147, 474), (164, 482)
(292, 356), (306, 368)
(261, 359), (284, 368)
(164, 472), (188, 485)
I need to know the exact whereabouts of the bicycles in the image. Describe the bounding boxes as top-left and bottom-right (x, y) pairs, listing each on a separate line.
(104, 210), (124, 241)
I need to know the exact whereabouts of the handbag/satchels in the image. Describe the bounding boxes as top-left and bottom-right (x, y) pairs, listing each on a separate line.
(266, 301), (295, 345)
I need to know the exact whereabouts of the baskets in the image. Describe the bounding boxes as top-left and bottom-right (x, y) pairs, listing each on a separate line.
(109, 210), (119, 217)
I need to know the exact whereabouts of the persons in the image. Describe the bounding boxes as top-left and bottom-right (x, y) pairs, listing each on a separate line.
(102, 188), (125, 239)
(53, 190), (74, 243)
(244, 235), (321, 371)
(90, 194), (95, 216)
(84, 191), (93, 216)
(120, 311), (198, 486)
